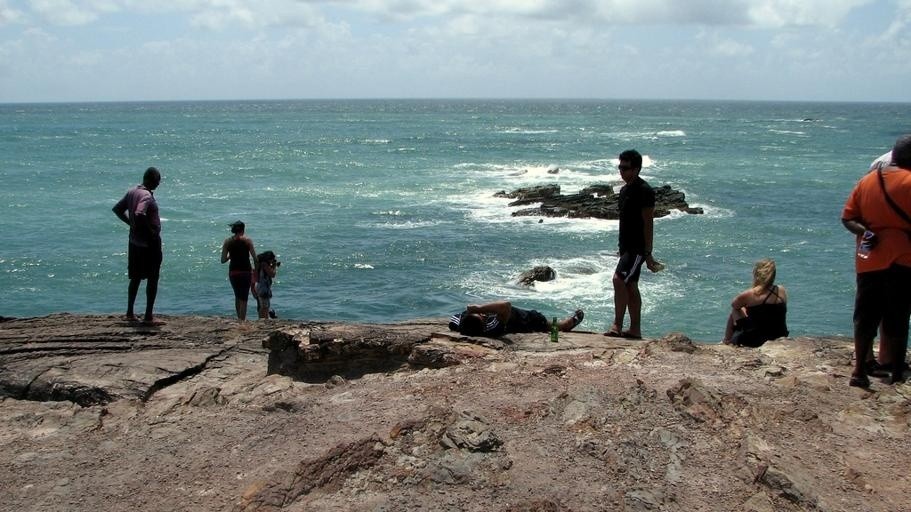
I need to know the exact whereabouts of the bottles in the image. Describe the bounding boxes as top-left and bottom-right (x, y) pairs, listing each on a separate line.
(858, 232), (874, 259)
(551, 316), (560, 341)
(655, 263), (666, 270)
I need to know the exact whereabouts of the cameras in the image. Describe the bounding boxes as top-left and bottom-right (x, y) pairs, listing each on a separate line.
(272, 260), (280, 267)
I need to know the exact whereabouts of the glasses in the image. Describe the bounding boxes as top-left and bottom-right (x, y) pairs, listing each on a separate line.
(618, 165), (632, 171)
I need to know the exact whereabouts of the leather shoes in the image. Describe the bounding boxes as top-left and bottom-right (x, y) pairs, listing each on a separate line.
(866, 359), (892, 378)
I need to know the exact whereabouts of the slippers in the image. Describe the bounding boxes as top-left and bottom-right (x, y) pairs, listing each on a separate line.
(610, 328), (619, 337)
(122, 316), (137, 320)
(144, 319), (167, 325)
(620, 332), (641, 339)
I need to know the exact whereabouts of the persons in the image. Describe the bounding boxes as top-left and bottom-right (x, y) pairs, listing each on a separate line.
(448, 300), (587, 339)
(863, 147), (909, 378)
(222, 219), (260, 321)
(840, 134), (911, 389)
(251, 265), (260, 315)
(722, 258), (790, 348)
(255, 250), (278, 321)
(604, 149), (664, 339)
(112, 166), (167, 327)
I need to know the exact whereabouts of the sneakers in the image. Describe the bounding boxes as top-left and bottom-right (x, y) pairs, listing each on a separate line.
(562, 310), (583, 331)
(850, 372), (869, 387)
